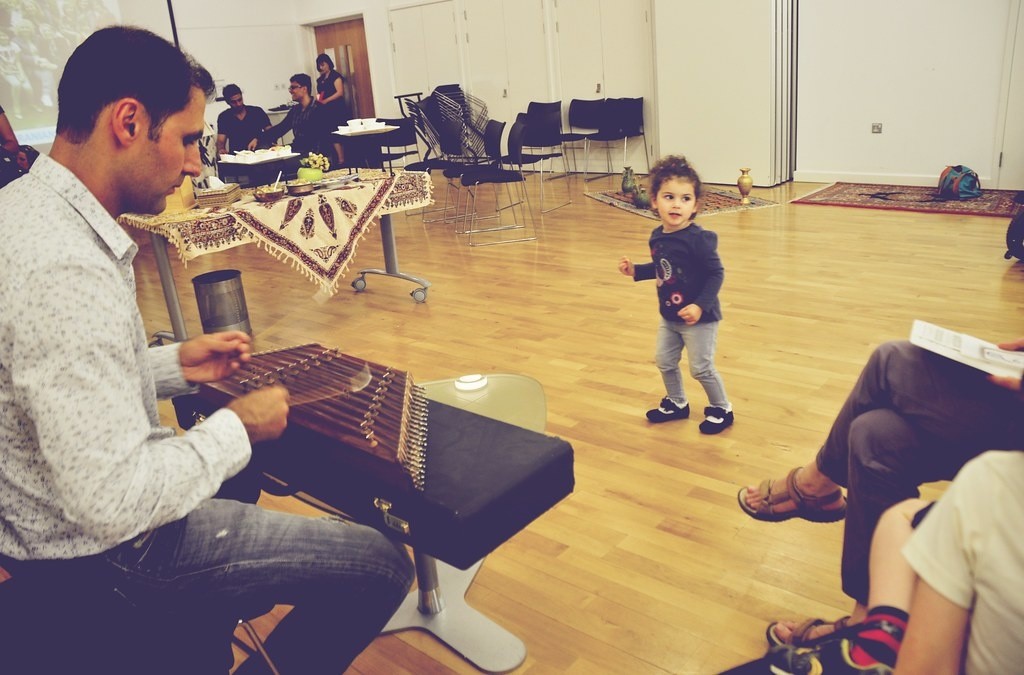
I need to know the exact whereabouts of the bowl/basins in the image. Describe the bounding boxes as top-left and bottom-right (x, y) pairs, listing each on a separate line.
(288, 183), (315, 195)
(252, 185), (285, 203)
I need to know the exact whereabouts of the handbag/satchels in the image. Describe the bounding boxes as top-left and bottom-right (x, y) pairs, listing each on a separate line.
(938, 165), (983, 200)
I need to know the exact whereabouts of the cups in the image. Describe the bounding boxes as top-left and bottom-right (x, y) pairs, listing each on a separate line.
(346, 116), (378, 129)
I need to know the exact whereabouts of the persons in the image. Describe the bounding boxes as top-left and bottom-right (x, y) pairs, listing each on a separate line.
(0, 105), (28, 170)
(738, 336), (1024, 675)
(0, 27), (415, 675)
(216, 84), (277, 187)
(316, 53), (352, 126)
(0, 0), (115, 127)
(248, 74), (344, 171)
(619, 156), (734, 434)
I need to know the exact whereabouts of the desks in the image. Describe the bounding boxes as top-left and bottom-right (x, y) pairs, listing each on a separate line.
(173, 392), (574, 673)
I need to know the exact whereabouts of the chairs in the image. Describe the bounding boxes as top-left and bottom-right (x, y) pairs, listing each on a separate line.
(376, 85), (644, 244)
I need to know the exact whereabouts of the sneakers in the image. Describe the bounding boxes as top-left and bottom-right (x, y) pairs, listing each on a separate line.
(764, 638), (893, 675)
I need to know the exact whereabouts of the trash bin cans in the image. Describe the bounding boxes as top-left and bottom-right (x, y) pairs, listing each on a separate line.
(191, 269), (252, 335)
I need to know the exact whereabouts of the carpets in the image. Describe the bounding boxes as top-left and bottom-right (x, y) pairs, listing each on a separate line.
(585, 182), (776, 220)
(790, 181), (1024, 218)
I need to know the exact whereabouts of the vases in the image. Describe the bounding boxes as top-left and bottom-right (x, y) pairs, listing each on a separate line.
(623, 162), (753, 206)
(297, 168), (322, 181)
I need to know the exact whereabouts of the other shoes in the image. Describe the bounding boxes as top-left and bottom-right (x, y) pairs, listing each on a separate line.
(645, 396), (690, 422)
(699, 407), (734, 434)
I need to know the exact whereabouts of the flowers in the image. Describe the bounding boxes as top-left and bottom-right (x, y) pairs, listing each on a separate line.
(304, 153), (331, 169)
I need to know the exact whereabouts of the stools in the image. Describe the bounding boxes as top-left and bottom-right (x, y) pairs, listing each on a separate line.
(0, 576), (279, 675)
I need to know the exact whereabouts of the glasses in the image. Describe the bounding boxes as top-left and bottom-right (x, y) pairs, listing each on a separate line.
(288, 85), (302, 91)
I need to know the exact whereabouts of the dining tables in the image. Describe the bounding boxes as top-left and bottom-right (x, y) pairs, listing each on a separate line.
(217, 152), (301, 184)
(127, 165), (431, 346)
(328, 130), (399, 170)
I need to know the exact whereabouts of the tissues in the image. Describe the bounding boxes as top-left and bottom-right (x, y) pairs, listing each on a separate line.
(196, 175), (243, 208)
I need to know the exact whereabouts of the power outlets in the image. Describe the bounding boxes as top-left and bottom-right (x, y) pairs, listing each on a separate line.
(873, 123), (881, 132)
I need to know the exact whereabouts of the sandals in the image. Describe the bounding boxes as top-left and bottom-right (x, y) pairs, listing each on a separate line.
(737, 467), (848, 522)
(766, 616), (850, 646)
